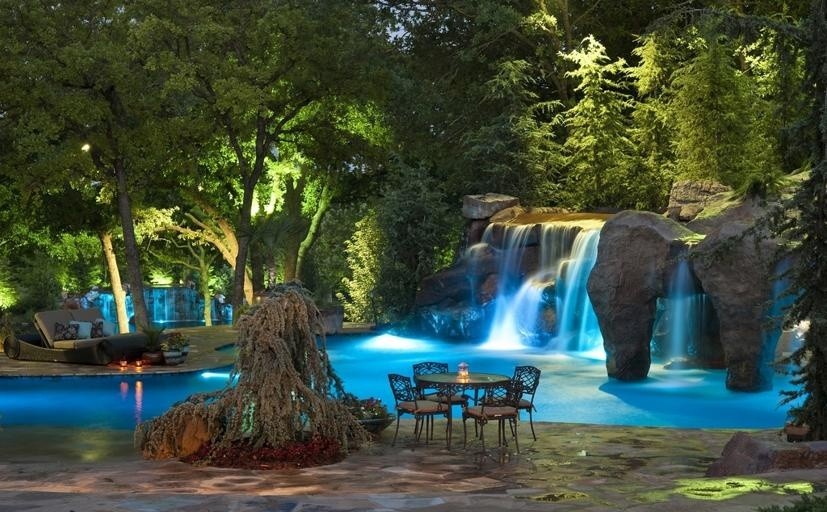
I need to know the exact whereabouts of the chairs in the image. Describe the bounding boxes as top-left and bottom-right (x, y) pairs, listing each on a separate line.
(388, 362), (541, 454)
(3, 307), (137, 361)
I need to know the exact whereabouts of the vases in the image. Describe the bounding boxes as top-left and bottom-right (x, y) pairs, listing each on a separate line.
(140, 346), (189, 365)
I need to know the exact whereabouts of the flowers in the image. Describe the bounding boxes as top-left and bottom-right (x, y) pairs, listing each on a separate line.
(137, 321), (190, 351)
